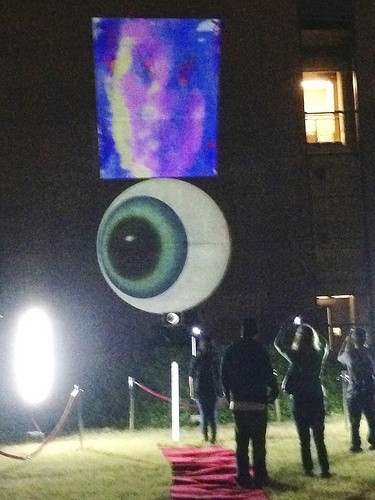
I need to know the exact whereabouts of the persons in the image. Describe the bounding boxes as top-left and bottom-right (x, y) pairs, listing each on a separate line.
(338, 327), (375, 453)
(274, 324), (332, 479)
(189, 336), (225, 445)
(220, 320), (279, 489)
